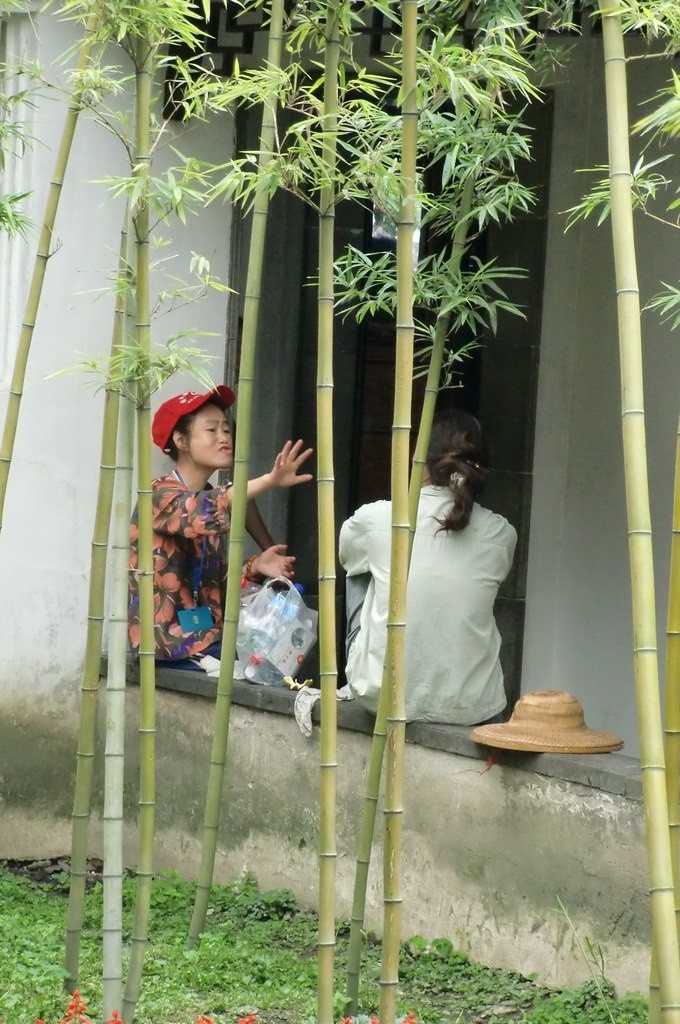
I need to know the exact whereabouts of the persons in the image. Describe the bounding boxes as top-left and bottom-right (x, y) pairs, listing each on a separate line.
(128, 385), (313, 671)
(340, 411), (519, 723)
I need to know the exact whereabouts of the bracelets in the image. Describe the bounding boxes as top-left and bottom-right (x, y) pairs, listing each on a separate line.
(247, 554), (260, 581)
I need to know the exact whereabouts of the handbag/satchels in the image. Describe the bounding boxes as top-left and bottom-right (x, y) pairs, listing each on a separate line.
(235, 576), (318, 688)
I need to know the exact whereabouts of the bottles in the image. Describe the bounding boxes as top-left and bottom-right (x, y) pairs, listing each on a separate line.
(238, 578), (315, 686)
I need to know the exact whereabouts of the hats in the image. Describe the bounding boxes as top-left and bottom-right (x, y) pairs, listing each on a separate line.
(469, 691), (625, 754)
(151, 384), (237, 455)
